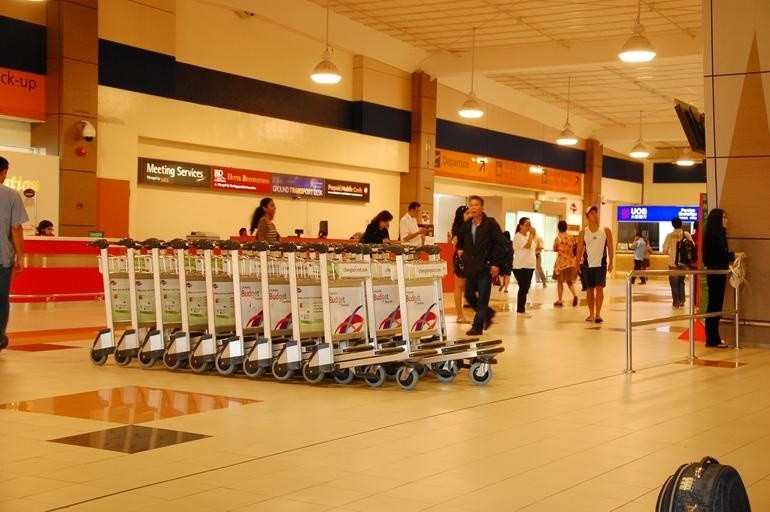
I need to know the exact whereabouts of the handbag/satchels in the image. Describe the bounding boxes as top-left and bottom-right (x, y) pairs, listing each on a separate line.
(729, 257), (746, 289)
(495, 231), (514, 276)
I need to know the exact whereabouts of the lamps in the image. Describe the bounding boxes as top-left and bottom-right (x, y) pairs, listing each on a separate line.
(311, 4), (345, 85)
(555, 75), (581, 146)
(628, 109), (652, 159)
(457, 27), (485, 120)
(617, 0), (655, 64)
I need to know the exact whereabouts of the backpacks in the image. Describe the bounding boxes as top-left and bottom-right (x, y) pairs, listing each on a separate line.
(675, 231), (697, 266)
(655, 457), (751, 512)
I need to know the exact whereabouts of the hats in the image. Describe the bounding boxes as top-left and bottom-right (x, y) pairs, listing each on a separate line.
(585, 206), (598, 217)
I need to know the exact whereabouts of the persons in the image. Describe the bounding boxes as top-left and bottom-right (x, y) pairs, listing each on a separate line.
(702, 209), (747, 349)
(35, 220), (55, 236)
(451, 196), (613, 336)
(631, 217), (697, 309)
(239, 198), (278, 244)
(399, 201), (427, 249)
(0, 156), (30, 350)
(359, 210), (393, 244)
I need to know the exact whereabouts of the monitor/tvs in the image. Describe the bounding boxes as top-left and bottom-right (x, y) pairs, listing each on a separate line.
(673, 103), (706, 154)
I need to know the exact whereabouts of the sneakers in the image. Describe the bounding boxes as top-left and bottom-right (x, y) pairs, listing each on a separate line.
(457, 309), (496, 336)
(517, 312), (532, 318)
(585, 317), (603, 323)
(554, 296), (578, 307)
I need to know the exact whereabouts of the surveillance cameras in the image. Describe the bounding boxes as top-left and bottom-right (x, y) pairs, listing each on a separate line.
(601, 195), (607, 205)
(83, 124), (97, 142)
